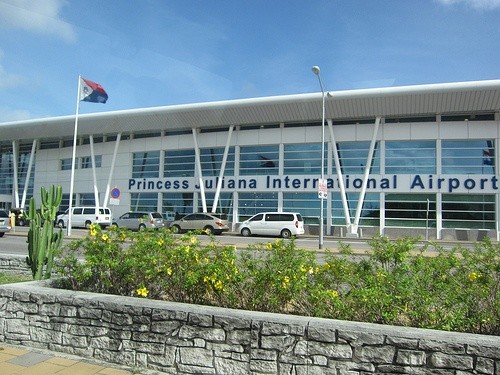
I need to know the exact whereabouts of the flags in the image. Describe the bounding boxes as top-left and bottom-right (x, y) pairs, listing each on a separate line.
(79, 77), (108, 104)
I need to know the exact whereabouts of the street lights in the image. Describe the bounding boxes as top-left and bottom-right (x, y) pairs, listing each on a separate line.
(311, 65), (326, 249)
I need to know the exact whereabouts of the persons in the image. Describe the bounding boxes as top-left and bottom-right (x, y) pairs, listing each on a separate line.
(175, 211), (178, 220)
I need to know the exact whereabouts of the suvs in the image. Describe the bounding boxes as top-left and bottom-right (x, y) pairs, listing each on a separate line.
(112, 211), (166, 233)
(0, 209), (12, 238)
(237, 211), (305, 239)
(55, 206), (113, 229)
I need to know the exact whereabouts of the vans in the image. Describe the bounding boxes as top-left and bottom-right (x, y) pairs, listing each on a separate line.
(169, 212), (230, 235)
